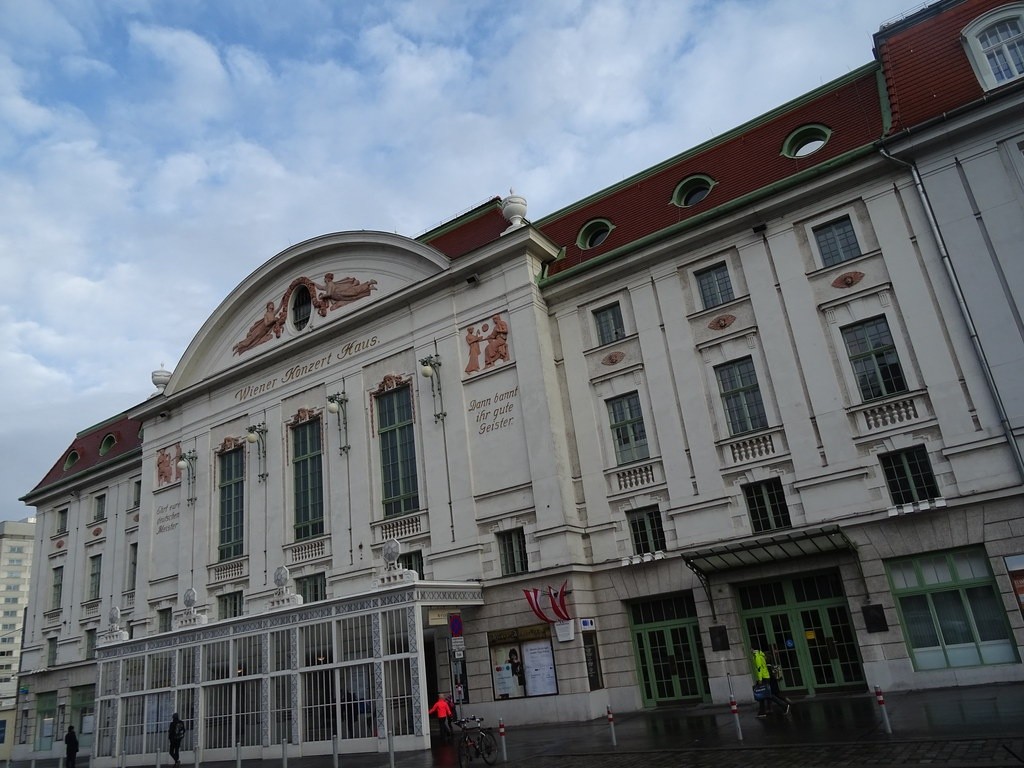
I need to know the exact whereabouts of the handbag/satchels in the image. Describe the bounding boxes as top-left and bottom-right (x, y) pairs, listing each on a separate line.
(752, 683), (768, 702)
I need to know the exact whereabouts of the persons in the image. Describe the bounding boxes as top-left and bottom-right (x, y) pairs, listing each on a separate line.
(429, 693), (457, 739)
(232, 293), (286, 356)
(750, 644), (791, 718)
(156, 443), (182, 484)
(64, 725), (79, 768)
(464, 315), (508, 375)
(166, 712), (186, 766)
(308, 273), (378, 310)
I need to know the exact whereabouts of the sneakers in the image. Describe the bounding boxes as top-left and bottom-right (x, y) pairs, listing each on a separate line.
(754, 713), (768, 719)
(783, 705), (790, 715)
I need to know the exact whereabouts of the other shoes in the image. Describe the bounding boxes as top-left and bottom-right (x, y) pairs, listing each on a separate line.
(174, 759), (181, 768)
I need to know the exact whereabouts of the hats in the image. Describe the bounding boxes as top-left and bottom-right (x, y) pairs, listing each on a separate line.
(438, 693), (445, 699)
(445, 693), (452, 703)
(172, 712), (180, 720)
(750, 642), (760, 650)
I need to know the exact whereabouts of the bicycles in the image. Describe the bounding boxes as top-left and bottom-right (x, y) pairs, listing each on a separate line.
(454, 715), (499, 768)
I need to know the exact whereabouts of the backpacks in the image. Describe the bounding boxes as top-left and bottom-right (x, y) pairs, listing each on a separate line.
(772, 665), (783, 682)
(175, 722), (184, 738)
